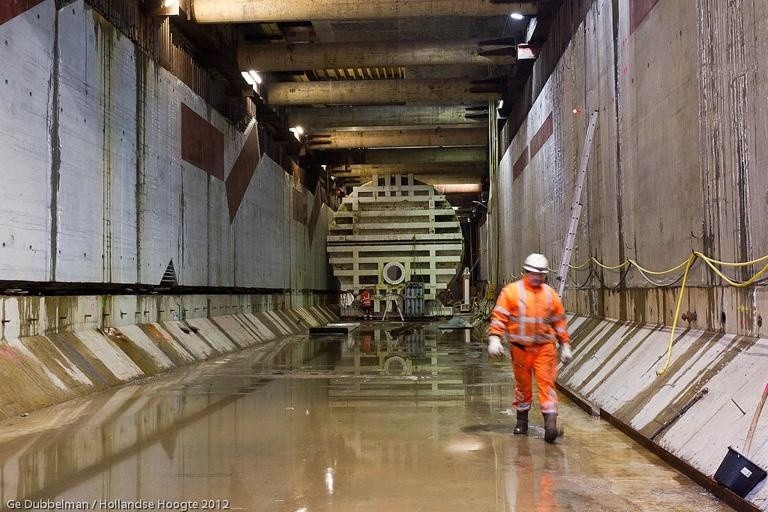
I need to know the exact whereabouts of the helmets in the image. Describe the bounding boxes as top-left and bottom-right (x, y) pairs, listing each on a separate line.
(522, 253), (549, 274)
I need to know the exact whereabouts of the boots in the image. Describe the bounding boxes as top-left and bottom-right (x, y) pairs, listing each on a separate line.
(542, 412), (565, 444)
(363, 315), (373, 321)
(512, 410), (529, 435)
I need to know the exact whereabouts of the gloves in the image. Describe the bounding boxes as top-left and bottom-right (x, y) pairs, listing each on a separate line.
(487, 335), (506, 361)
(560, 342), (574, 366)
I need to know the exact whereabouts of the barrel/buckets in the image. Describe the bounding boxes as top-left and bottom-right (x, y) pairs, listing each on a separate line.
(713, 438), (768, 498)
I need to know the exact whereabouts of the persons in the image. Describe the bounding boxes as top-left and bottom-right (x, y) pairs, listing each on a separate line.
(359, 289), (374, 322)
(486, 252), (574, 444)
(509, 440), (563, 511)
